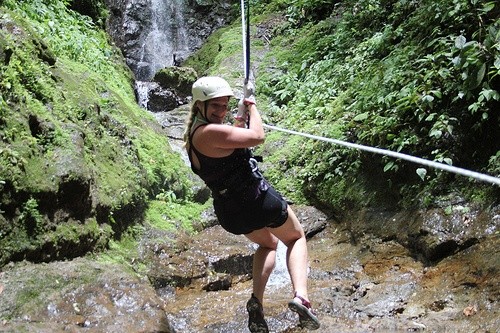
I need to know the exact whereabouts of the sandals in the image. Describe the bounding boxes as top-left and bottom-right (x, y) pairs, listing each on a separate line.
(246, 293), (269, 333)
(286, 291), (320, 330)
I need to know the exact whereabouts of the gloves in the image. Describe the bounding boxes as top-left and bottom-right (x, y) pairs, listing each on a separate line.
(243, 80), (256, 107)
(235, 96), (247, 121)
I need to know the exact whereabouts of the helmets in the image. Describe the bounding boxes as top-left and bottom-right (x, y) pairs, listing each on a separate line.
(191, 75), (234, 105)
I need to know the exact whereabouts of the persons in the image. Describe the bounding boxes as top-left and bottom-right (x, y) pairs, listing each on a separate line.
(184, 75), (321, 333)
